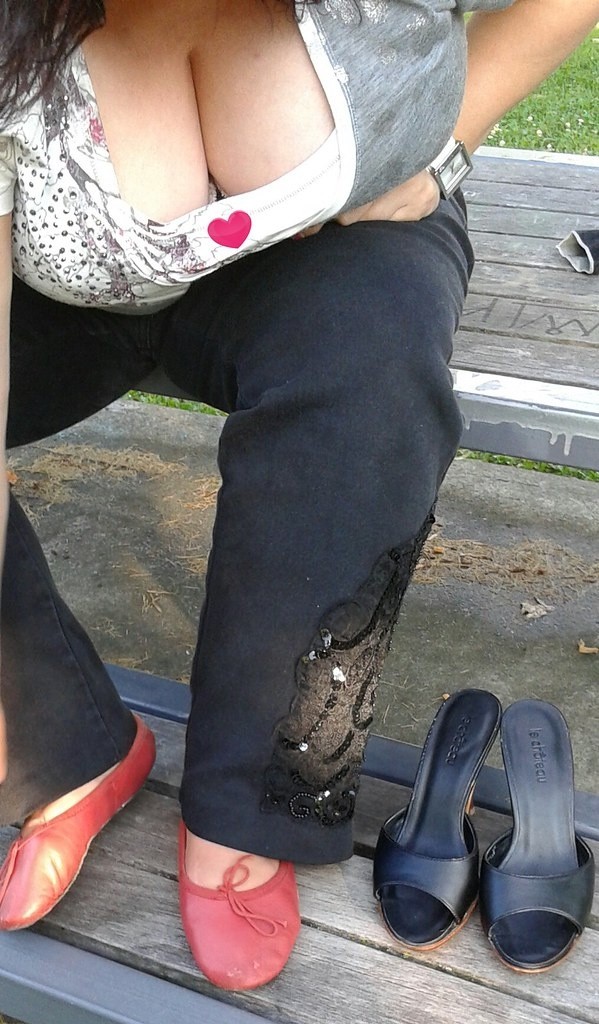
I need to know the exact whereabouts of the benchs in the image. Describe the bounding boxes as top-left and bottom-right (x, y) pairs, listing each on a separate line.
(0, 666), (599, 1024)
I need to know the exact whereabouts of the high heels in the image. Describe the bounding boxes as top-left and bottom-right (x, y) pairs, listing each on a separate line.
(372, 687), (502, 953)
(478, 699), (596, 974)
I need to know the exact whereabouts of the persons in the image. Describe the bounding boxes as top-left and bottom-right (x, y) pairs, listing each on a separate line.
(0, 1), (596, 997)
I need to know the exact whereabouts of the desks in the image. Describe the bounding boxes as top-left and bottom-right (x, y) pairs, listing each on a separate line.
(444, 137), (599, 494)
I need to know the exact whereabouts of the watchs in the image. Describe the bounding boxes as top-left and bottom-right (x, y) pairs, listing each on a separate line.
(425, 134), (475, 202)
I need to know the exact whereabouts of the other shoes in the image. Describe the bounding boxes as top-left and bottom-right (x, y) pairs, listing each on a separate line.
(176, 816), (302, 991)
(0, 713), (157, 931)
